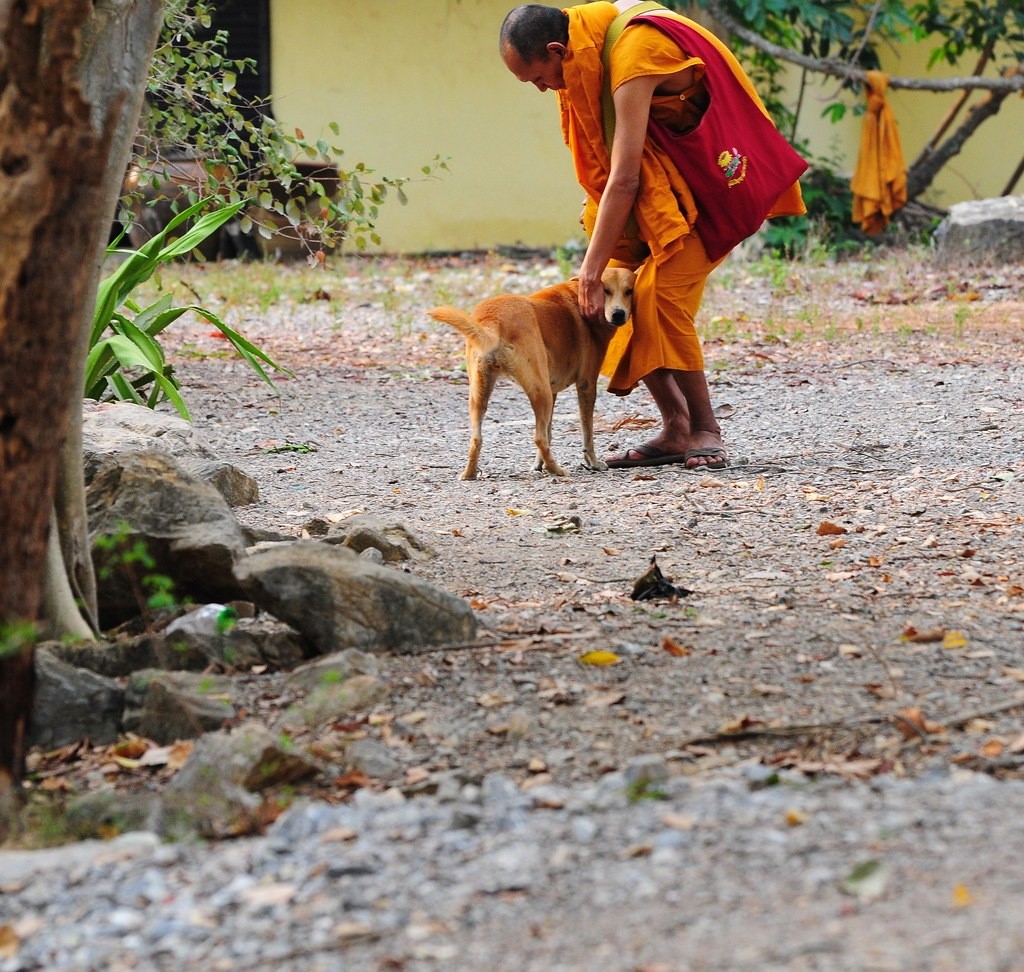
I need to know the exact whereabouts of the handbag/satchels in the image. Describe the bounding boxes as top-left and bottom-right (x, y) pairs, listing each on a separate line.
(621, 15), (809, 263)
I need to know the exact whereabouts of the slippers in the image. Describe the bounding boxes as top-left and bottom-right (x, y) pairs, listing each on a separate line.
(602, 443), (730, 470)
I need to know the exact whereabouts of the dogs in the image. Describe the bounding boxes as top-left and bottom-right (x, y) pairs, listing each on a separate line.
(425, 268), (639, 481)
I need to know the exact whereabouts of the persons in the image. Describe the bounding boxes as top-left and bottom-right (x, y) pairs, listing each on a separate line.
(496, 0), (810, 472)
